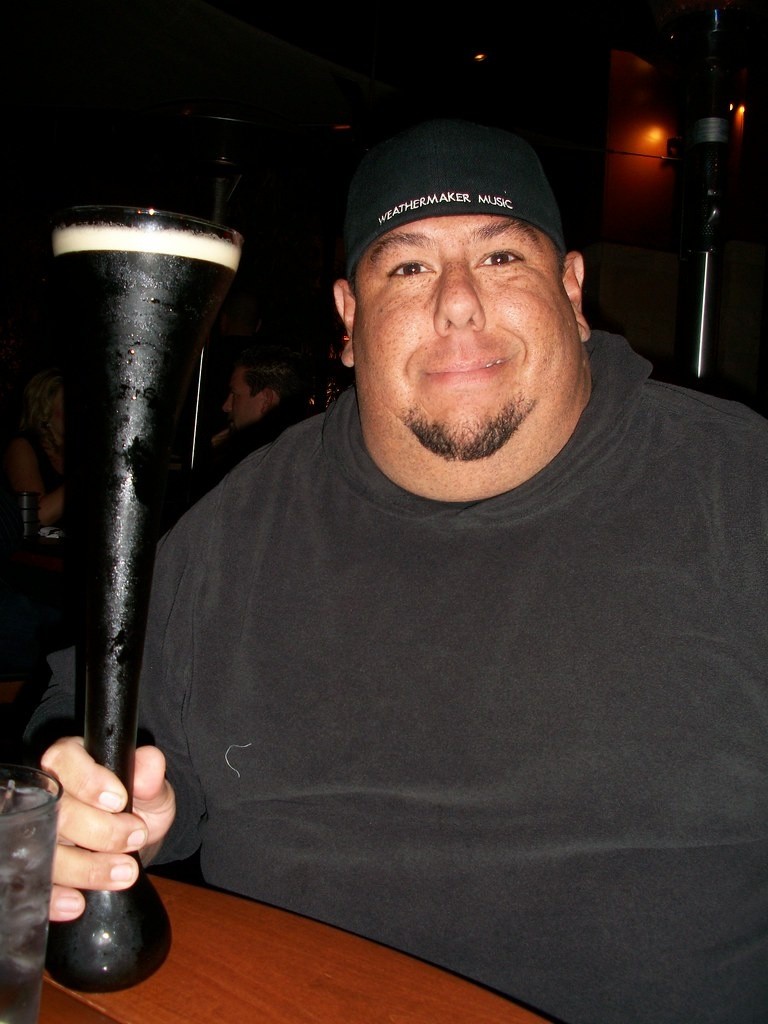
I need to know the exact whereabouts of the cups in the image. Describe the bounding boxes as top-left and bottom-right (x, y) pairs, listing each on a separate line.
(0, 760), (64, 1024)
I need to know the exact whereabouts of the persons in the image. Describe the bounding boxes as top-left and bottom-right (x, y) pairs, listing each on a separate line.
(138, 351), (323, 544)
(0, 123), (767, 1024)
(1, 372), (65, 764)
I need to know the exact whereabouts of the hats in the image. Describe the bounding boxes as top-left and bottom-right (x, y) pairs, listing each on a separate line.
(344, 118), (568, 285)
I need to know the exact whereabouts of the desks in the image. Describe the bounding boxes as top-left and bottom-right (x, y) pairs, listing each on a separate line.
(39, 872), (552, 1023)
